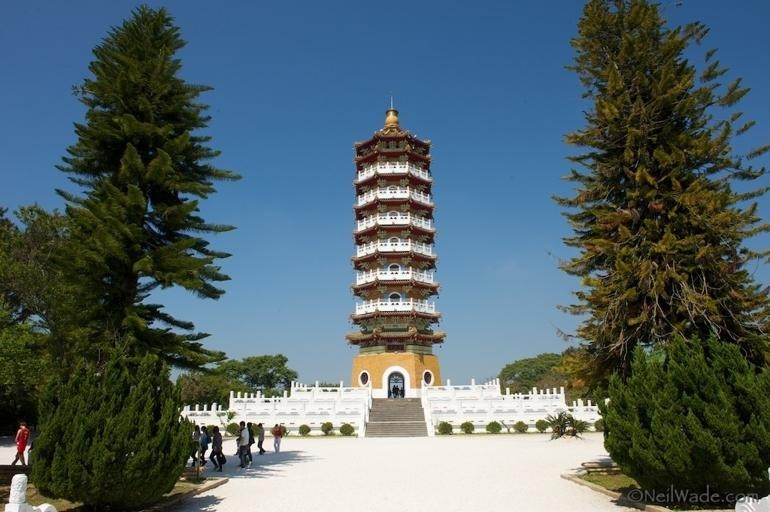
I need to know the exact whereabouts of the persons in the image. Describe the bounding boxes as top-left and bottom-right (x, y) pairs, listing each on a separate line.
(200, 425), (208, 465)
(209, 425), (222, 472)
(271, 423), (281, 453)
(255, 422), (266, 455)
(245, 421), (255, 466)
(190, 425), (201, 466)
(10, 419), (31, 467)
(388, 384), (404, 398)
(237, 420), (250, 471)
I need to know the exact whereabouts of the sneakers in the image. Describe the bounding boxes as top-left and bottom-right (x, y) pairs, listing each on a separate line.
(191, 449), (266, 473)
